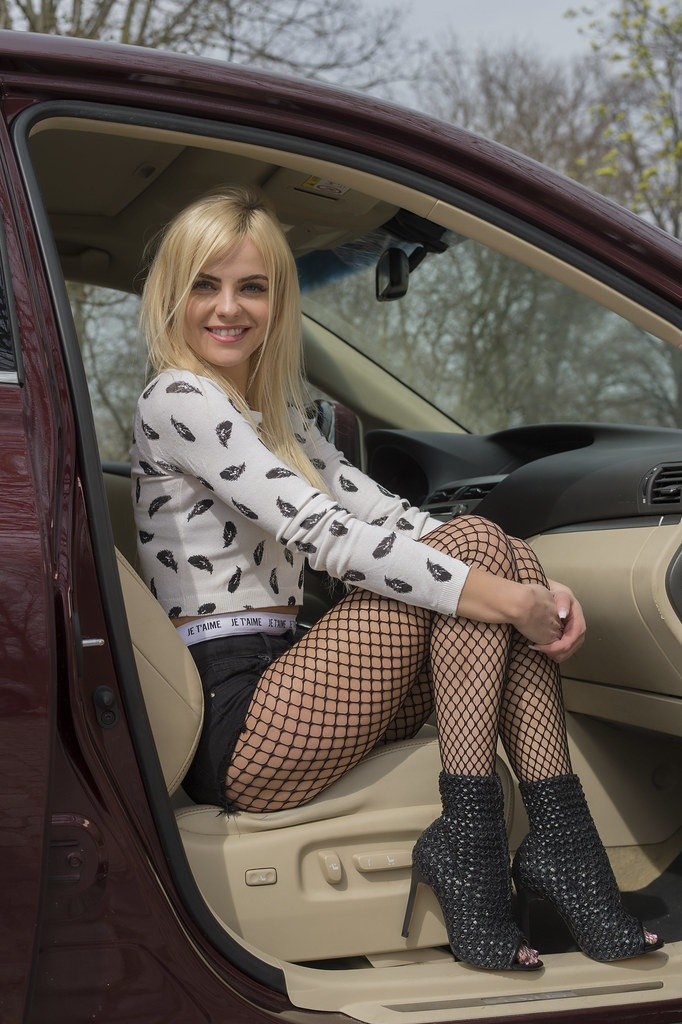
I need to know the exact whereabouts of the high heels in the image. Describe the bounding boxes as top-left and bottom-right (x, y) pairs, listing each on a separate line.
(401, 771), (544, 971)
(511, 773), (665, 963)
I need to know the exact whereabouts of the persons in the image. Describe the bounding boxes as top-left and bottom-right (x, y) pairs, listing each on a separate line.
(128, 194), (664, 971)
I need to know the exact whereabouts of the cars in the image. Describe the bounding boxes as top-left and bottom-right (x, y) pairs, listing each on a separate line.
(0, 26), (682, 1024)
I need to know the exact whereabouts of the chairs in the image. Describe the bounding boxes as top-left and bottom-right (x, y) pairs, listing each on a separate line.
(115, 544), (515, 964)
